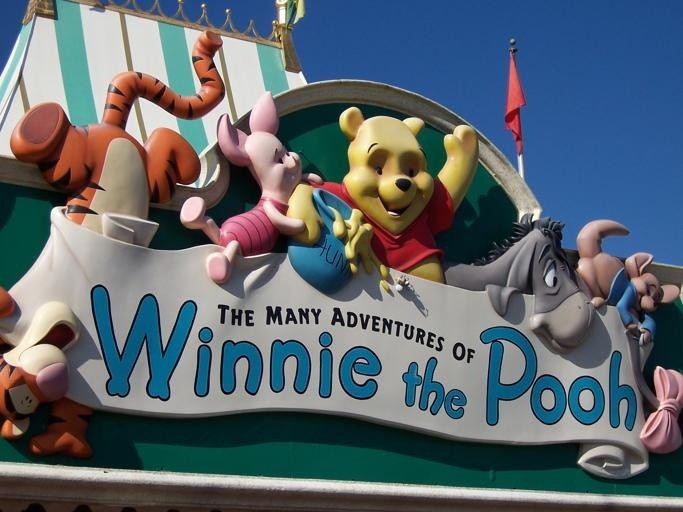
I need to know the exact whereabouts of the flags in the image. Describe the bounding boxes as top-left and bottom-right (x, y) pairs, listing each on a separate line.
(505, 50), (527, 155)
